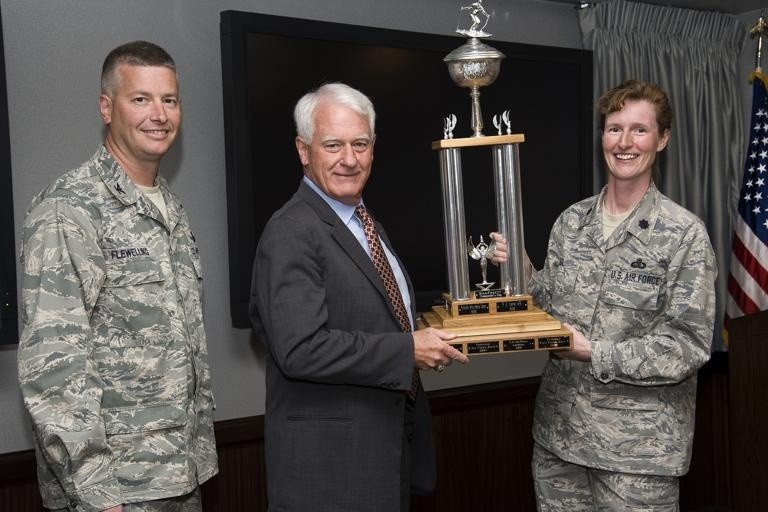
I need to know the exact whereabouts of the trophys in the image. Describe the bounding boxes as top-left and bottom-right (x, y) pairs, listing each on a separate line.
(413, 1), (575, 397)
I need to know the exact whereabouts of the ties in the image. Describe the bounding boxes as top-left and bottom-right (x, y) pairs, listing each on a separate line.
(355, 206), (420, 401)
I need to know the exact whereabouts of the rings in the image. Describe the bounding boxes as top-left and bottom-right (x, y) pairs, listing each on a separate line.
(436, 365), (447, 373)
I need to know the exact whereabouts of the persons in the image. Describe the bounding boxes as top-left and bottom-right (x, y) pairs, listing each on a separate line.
(16, 41), (223, 512)
(246, 82), (471, 511)
(486, 79), (719, 512)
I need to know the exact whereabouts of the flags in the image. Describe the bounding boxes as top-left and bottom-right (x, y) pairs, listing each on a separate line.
(719, 68), (768, 350)
